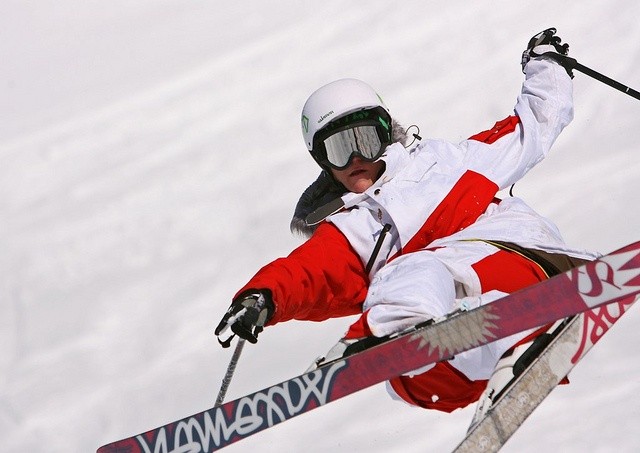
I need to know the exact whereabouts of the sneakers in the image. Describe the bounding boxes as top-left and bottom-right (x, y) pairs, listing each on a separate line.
(304, 320), (431, 373)
(468, 318), (572, 433)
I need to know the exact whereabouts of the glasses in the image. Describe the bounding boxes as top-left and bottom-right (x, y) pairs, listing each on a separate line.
(317, 120), (385, 170)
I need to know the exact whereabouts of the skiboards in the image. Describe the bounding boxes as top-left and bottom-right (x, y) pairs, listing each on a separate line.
(97, 241), (640, 453)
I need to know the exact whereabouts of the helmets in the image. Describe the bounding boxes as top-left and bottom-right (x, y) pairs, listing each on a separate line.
(301, 79), (392, 172)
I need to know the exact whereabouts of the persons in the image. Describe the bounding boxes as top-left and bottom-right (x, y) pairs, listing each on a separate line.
(214, 27), (602, 433)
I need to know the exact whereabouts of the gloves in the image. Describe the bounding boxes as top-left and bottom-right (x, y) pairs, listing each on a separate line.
(522, 27), (575, 80)
(215, 289), (276, 348)
